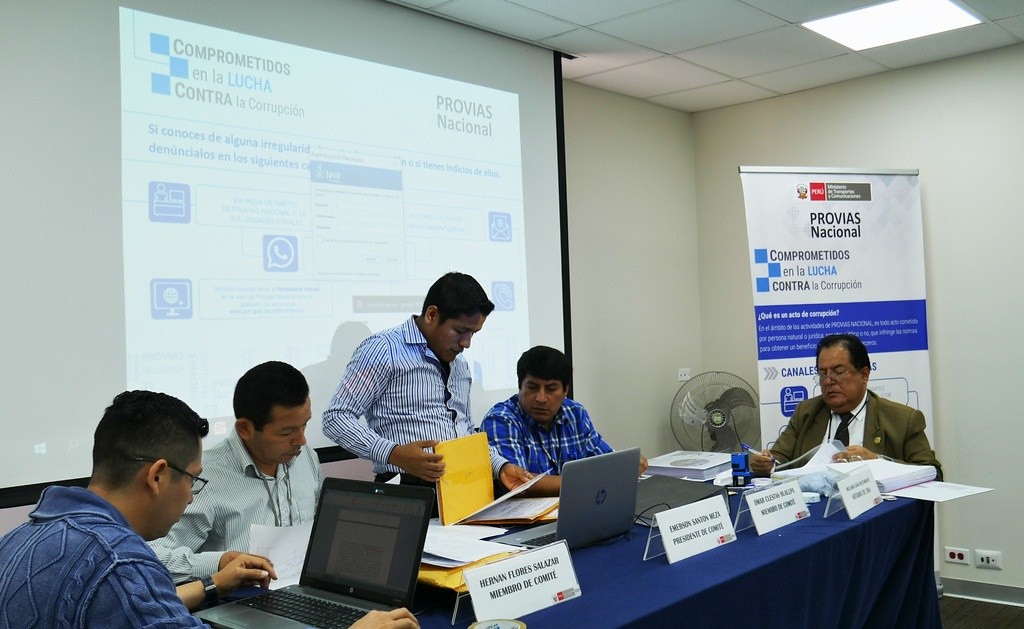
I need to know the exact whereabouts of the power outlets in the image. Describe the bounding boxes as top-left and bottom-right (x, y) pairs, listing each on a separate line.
(944, 547), (970, 565)
(679, 368), (692, 381)
(974, 550), (1003, 570)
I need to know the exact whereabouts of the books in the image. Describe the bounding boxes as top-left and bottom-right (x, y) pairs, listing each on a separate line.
(647, 450), (732, 479)
(770, 444), (937, 491)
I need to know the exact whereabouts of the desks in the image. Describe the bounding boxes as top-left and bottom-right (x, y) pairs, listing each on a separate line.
(175, 471), (943, 629)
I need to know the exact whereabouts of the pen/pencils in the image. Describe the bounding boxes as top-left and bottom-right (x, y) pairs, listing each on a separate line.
(741, 443), (782, 465)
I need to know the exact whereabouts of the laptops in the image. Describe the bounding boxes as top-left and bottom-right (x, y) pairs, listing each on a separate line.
(488, 447), (640, 549)
(191, 477), (435, 628)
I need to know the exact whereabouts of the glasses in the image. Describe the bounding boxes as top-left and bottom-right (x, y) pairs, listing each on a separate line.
(812, 366), (867, 386)
(132, 454), (209, 495)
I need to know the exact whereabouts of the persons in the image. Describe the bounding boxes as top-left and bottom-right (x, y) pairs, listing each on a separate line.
(480, 345), (648, 488)
(0, 390), (420, 629)
(321, 271), (535, 494)
(144, 361), (321, 585)
(751, 333), (942, 482)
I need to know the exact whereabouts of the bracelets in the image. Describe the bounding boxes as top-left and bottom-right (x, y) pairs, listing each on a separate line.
(200, 575), (218, 604)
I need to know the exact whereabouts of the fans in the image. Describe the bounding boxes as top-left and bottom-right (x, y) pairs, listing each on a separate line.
(670, 371), (761, 453)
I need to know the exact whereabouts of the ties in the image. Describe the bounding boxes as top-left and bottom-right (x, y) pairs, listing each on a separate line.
(834, 412), (854, 447)
